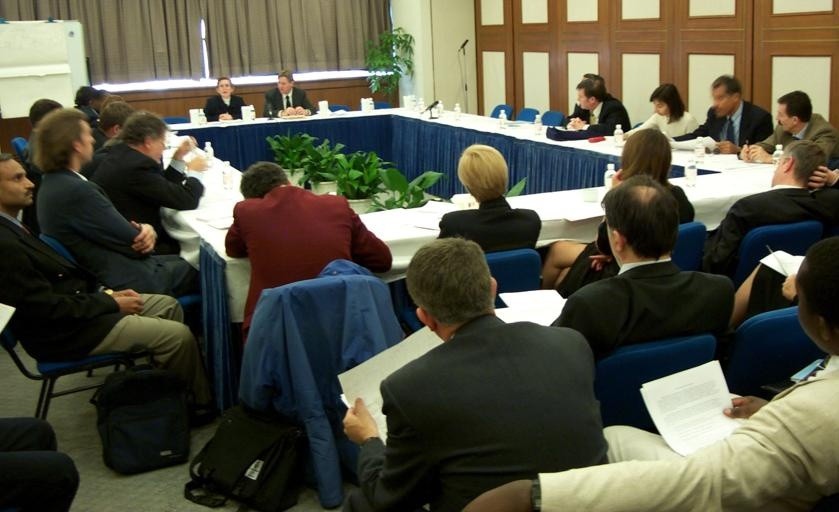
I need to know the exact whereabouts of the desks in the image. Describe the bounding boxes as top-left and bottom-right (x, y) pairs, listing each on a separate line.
(161, 104), (683, 413)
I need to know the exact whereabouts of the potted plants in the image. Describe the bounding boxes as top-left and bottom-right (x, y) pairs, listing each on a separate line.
(291, 138), (345, 196)
(328, 150), (394, 217)
(266, 133), (317, 188)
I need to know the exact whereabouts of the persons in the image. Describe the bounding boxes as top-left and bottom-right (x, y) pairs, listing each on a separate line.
(91, 102), (209, 174)
(624, 83), (698, 140)
(23, 99), (63, 171)
(1, 153), (211, 425)
(437, 144), (541, 256)
(742, 91), (839, 166)
(546, 175), (734, 361)
(89, 111), (207, 256)
(31, 107), (196, 298)
(669, 75), (774, 154)
(263, 71), (316, 118)
(74, 86), (124, 129)
(705, 140), (839, 277)
(342, 237), (609, 512)
(730, 259), (798, 330)
(562, 74), (632, 134)
(224, 162), (392, 347)
(203, 77), (245, 122)
(460, 236), (839, 512)
(542, 128), (695, 298)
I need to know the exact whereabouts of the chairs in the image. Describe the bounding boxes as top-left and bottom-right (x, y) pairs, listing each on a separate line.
(672, 222), (707, 270)
(374, 102), (392, 109)
(490, 104), (513, 120)
(314, 104), (351, 112)
(0, 317), (151, 419)
(515, 108), (539, 123)
(541, 111), (565, 126)
(730, 305), (829, 399)
(394, 249), (543, 331)
(593, 334), (715, 438)
(12, 137), (29, 166)
(230, 271), (387, 423)
(39, 234), (203, 310)
(731, 220), (821, 284)
(162, 116), (190, 124)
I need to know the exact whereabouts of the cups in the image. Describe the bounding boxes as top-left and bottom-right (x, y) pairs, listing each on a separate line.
(361, 99), (370, 113)
(241, 106), (250, 120)
(403, 94), (415, 113)
(318, 101), (330, 114)
(188, 109), (199, 123)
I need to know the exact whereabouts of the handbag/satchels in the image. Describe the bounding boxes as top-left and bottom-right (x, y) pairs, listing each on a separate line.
(183, 405), (302, 512)
(90, 362), (191, 475)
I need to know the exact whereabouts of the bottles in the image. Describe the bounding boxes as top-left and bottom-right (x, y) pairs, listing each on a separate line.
(684, 158), (697, 188)
(498, 110), (507, 128)
(411, 94), (416, 108)
(614, 124), (624, 147)
(533, 114), (542, 135)
(419, 98), (425, 111)
(368, 97), (374, 114)
(222, 159), (234, 190)
(249, 104), (256, 121)
(198, 108), (206, 126)
(203, 141), (215, 173)
(453, 103), (461, 120)
(771, 144), (784, 174)
(603, 164), (616, 190)
(694, 136), (706, 168)
(436, 100), (444, 118)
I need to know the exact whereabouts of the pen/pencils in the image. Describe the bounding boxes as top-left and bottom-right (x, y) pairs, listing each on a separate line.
(746, 139), (749, 148)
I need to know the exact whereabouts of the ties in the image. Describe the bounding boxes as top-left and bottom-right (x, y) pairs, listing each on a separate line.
(286, 96), (291, 108)
(726, 118), (735, 144)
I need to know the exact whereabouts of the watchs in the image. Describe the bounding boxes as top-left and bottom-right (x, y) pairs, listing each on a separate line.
(531, 479), (541, 511)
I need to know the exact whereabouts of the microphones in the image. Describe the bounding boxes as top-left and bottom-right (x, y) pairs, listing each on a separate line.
(267, 104), (274, 121)
(457, 40), (469, 54)
(420, 101), (441, 119)
